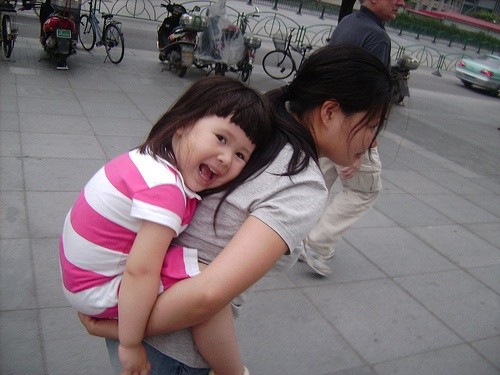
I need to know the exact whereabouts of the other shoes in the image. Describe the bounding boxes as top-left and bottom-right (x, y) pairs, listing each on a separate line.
(296, 243), (335, 261)
(301, 236), (332, 277)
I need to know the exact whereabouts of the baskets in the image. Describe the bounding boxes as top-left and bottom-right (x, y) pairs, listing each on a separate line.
(272, 32), (288, 51)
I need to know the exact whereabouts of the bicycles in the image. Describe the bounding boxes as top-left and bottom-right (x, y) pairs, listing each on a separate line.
(79, 0), (125, 64)
(0, 0), (46, 62)
(262, 28), (313, 80)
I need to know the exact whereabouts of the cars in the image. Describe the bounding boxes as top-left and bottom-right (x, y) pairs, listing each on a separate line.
(455, 53), (500, 98)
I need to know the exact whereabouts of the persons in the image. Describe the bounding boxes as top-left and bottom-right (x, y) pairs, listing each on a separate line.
(77, 45), (397, 374)
(298, 0), (406, 276)
(57, 77), (252, 374)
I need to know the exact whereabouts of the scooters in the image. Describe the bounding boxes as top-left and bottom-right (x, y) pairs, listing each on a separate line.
(157, 0), (208, 78)
(39, 0), (84, 67)
(192, 7), (262, 85)
